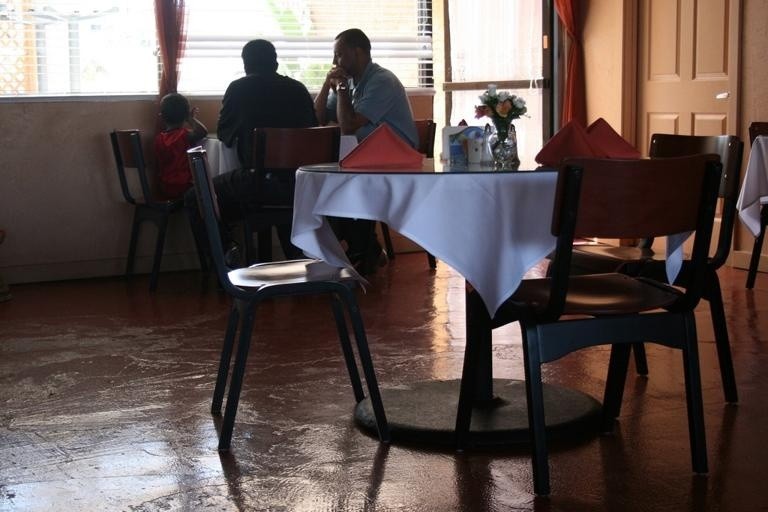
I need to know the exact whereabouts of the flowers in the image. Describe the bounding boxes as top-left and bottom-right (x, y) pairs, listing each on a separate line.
(474, 84), (527, 148)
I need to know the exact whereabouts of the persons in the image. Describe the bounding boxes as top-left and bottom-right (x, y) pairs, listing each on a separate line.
(311, 28), (421, 276)
(181, 38), (320, 267)
(145, 91), (208, 199)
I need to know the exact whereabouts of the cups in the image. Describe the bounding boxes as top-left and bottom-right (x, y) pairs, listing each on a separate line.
(468, 138), (482, 164)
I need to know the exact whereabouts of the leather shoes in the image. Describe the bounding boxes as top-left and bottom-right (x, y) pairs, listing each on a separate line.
(354, 250), (388, 275)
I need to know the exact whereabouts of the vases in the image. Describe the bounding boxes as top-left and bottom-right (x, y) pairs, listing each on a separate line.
(490, 131), (519, 163)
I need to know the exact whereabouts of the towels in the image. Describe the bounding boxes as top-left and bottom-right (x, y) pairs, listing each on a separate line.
(535, 119), (609, 167)
(340, 121), (423, 168)
(587, 118), (640, 159)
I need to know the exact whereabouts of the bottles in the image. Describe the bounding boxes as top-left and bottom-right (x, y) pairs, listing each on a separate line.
(450, 144), (463, 167)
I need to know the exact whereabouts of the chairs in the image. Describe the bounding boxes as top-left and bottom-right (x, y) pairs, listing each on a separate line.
(746, 122), (768, 289)
(110, 129), (208, 292)
(455, 153), (723, 494)
(186, 145), (391, 450)
(381, 119), (436, 267)
(245, 125), (341, 265)
(546, 133), (744, 419)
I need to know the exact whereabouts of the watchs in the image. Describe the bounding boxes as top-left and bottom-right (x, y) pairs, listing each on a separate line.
(335, 81), (349, 93)
